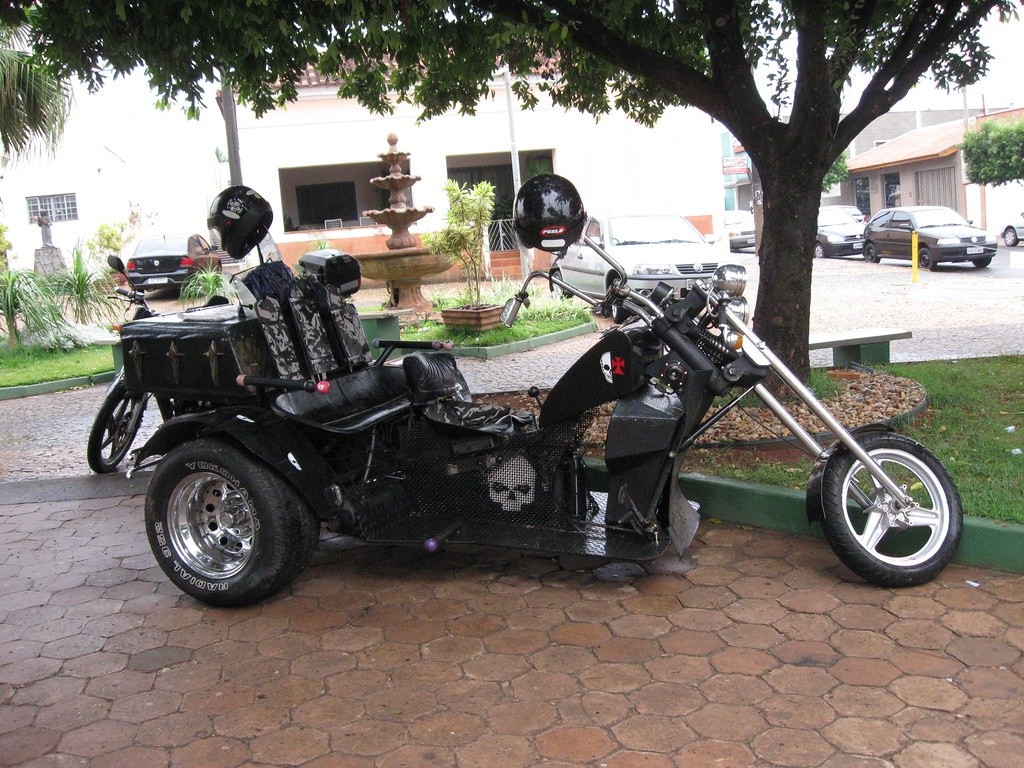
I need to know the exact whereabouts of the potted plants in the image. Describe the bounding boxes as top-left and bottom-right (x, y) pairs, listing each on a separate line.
(419, 178), (506, 336)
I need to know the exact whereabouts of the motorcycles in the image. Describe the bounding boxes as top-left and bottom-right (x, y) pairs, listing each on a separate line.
(116, 174), (967, 613)
(80, 246), (364, 474)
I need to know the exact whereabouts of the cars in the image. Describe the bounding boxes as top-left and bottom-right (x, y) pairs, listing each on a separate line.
(841, 202), (867, 225)
(125, 230), (205, 293)
(723, 208), (759, 250)
(810, 205), (866, 259)
(857, 204), (998, 271)
(1002, 207), (1024, 247)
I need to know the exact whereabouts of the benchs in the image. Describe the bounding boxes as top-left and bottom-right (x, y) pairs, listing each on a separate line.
(236, 260), (416, 435)
(806, 326), (914, 370)
(356, 309), (415, 361)
